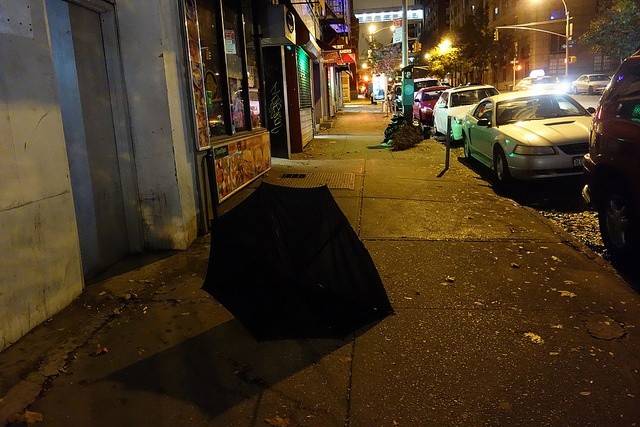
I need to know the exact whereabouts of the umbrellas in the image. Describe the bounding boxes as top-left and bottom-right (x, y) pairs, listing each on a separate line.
(198, 180), (394, 340)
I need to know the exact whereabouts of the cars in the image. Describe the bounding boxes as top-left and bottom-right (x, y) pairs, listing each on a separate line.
(231, 89), (260, 115)
(413, 85), (451, 124)
(461, 88), (595, 185)
(512, 69), (568, 90)
(570, 73), (612, 94)
(433, 84), (501, 140)
(389, 83), (403, 110)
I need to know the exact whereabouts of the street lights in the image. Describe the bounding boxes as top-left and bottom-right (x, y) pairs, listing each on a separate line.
(513, 58), (522, 87)
(371, 25), (396, 43)
(531, 0), (570, 76)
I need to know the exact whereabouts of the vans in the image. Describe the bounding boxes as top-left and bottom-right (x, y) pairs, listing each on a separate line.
(413, 77), (443, 119)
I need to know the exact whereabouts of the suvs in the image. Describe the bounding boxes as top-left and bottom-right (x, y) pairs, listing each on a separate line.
(581, 48), (640, 259)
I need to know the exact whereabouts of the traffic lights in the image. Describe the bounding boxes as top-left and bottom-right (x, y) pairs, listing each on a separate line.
(494, 30), (498, 41)
(417, 42), (422, 51)
